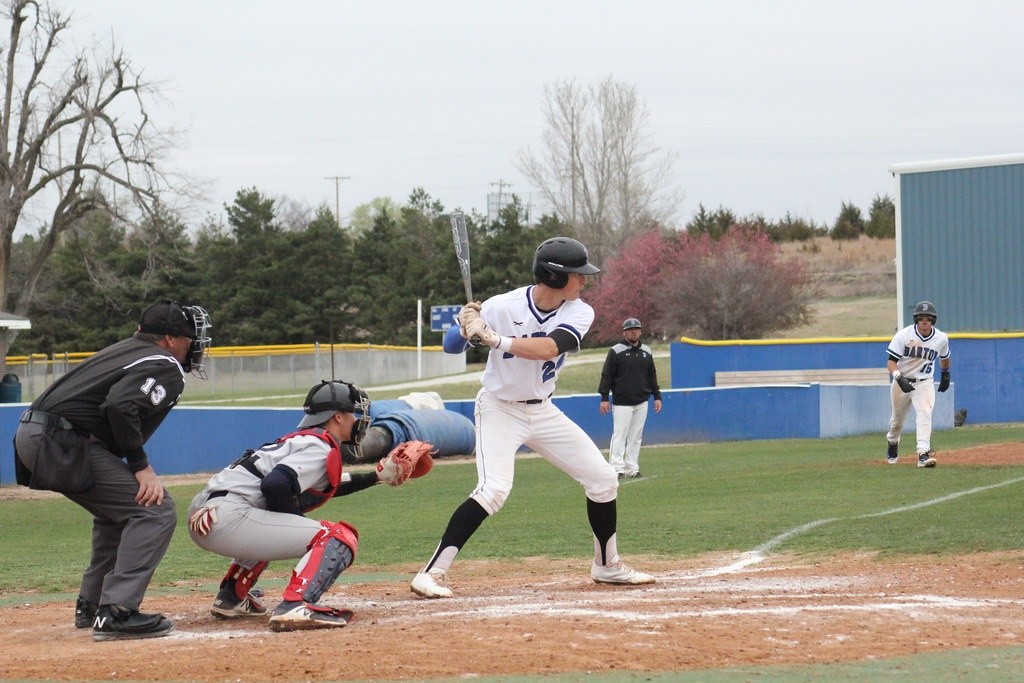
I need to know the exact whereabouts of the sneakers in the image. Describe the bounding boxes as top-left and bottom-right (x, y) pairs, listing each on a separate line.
(591, 556), (655, 584)
(887, 441), (899, 464)
(269, 601), (347, 631)
(91, 605), (175, 642)
(410, 571), (454, 598)
(917, 445), (938, 466)
(74, 599), (99, 628)
(210, 578), (269, 619)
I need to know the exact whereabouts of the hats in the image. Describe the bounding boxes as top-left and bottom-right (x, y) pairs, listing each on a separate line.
(623, 318), (643, 330)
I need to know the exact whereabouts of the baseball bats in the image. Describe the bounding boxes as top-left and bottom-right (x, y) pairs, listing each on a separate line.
(449, 211), (482, 346)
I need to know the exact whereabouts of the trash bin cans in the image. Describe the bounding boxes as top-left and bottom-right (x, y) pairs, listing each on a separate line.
(0, 374), (22, 403)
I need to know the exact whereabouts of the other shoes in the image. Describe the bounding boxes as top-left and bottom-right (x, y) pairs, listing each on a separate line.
(617, 472), (623, 479)
(633, 472), (641, 478)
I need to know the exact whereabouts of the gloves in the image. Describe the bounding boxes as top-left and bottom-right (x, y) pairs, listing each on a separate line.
(937, 369), (950, 393)
(895, 374), (916, 393)
(465, 318), (499, 347)
(458, 301), (482, 332)
(190, 505), (218, 536)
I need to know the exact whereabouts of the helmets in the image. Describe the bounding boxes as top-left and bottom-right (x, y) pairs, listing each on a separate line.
(913, 301), (937, 322)
(138, 299), (196, 338)
(297, 379), (360, 429)
(532, 237), (601, 289)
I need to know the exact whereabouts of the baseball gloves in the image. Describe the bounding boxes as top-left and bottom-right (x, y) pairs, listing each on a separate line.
(374, 438), (440, 488)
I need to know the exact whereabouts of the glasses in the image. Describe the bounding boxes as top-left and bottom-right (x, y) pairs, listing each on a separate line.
(916, 318), (934, 322)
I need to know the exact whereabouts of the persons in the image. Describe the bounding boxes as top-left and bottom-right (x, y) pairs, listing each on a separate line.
(598, 318), (663, 481)
(409, 237), (656, 598)
(886, 301), (951, 467)
(14, 298), (209, 641)
(187, 381), (433, 633)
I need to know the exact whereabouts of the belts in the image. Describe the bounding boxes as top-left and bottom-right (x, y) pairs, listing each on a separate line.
(206, 490), (228, 500)
(21, 411), (90, 437)
(910, 379), (926, 382)
(517, 394), (553, 404)
